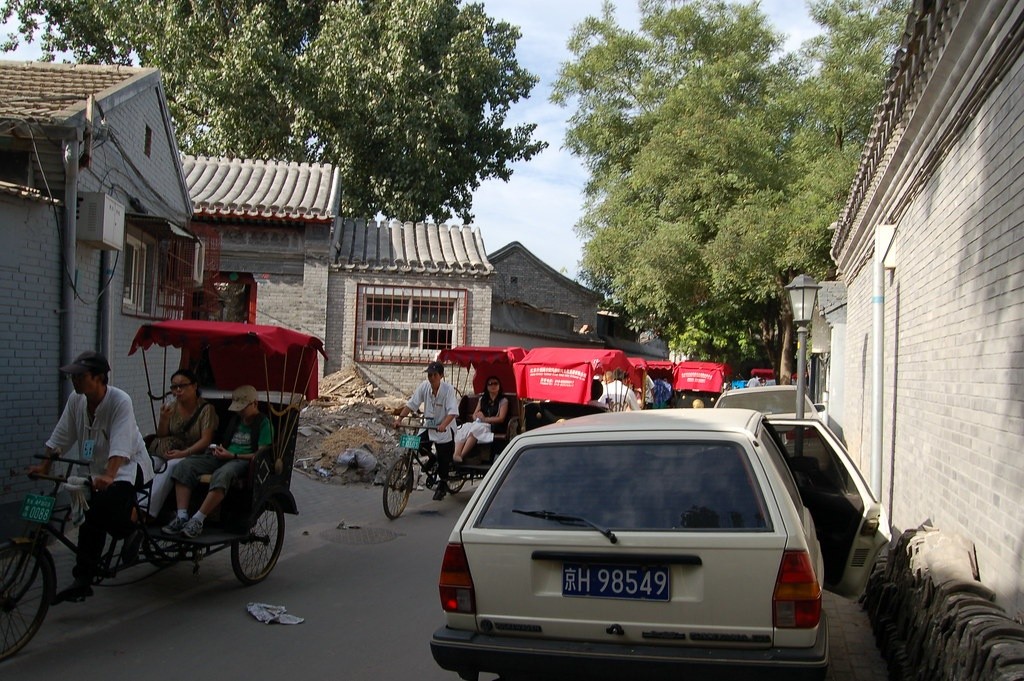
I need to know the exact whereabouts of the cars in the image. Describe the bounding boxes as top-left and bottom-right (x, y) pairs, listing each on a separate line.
(427, 407), (880, 681)
(714, 385), (826, 460)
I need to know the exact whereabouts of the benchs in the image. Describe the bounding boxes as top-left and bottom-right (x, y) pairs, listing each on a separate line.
(458, 393), (522, 447)
(142, 390), (304, 493)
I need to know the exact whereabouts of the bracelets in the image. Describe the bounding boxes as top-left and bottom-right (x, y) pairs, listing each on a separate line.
(234, 453), (240, 459)
(188, 447), (192, 455)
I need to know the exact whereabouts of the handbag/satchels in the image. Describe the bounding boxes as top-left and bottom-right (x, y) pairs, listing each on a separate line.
(147, 432), (186, 459)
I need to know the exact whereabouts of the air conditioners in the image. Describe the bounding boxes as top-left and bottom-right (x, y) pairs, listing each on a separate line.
(173, 235), (204, 286)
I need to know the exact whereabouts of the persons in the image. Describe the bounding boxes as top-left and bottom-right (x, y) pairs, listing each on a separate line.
(161, 385), (274, 538)
(131, 370), (221, 523)
(592, 365), (675, 412)
(721, 370), (810, 392)
(26, 350), (156, 603)
(393, 362), (459, 500)
(453, 376), (510, 463)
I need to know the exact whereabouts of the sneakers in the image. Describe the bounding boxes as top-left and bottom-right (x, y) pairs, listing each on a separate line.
(433, 481), (450, 500)
(420, 454), (437, 472)
(179, 517), (204, 539)
(161, 514), (190, 535)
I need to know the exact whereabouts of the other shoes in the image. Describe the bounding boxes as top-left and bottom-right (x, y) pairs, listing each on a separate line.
(121, 529), (144, 565)
(453, 453), (463, 463)
(50, 579), (94, 606)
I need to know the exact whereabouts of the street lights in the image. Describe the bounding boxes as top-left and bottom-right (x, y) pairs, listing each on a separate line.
(786, 273), (824, 472)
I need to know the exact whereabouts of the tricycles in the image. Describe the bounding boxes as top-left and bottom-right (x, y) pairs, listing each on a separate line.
(515, 345), (730, 429)
(751, 368), (776, 385)
(383, 346), (531, 520)
(0, 317), (324, 662)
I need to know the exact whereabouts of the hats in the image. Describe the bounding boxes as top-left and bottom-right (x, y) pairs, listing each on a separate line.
(60, 350), (110, 376)
(227, 385), (258, 412)
(425, 362), (444, 372)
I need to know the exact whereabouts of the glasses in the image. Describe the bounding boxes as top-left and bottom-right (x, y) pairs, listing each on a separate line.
(170, 381), (195, 391)
(487, 381), (499, 385)
